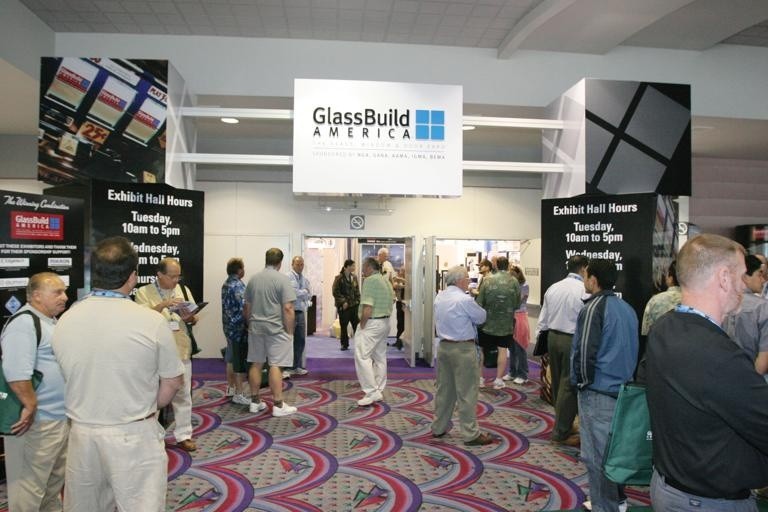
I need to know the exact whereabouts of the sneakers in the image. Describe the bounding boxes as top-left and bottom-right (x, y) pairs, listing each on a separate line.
(357, 391), (383, 406)
(249, 401), (267, 413)
(226, 386), (250, 405)
(493, 372), (529, 390)
(584, 499), (628, 512)
(464, 432), (492, 445)
(287, 367), (310, 375)
(272, 402), (298, 416)
(282, 370), (291, 380)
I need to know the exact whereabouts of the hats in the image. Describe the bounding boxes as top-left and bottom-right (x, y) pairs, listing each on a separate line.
(476, 260), (492, 266)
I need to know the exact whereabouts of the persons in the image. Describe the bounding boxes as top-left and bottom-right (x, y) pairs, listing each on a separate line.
(47, 234), (185, 512)
(134, 256), (202, 451)
(331, 259), (361, 352)
(640, 259), (683, 335)
(243, 247), (297, 417)
(353, 256), (394, 406)
(429, 266), (492, 444)
(377, 247), (394, 289)
(720, 254), (768, 375)
(532, 254), (592, 444)
(392, 263), (409, 348)
(468, 255), (531, 390)
(570, 255), (639, 512)
(643, 232), (768, 512)
(0, 271), (71, 512)
(221, 257), (251, 406)
(280, 256), (312, 379)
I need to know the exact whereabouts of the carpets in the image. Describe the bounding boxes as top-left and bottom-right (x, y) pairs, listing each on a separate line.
(1, 352), (662, 512)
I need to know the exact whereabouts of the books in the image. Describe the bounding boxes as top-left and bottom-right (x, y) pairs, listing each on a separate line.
(170, 301), (209, 314)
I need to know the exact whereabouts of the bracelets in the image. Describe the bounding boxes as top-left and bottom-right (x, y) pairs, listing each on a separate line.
(304, 287), (310, 294)
(186, 318), (194, 325)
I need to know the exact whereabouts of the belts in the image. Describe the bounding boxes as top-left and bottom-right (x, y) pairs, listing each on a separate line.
(369, 315), (390, 319)
(661, 474), (725, 499)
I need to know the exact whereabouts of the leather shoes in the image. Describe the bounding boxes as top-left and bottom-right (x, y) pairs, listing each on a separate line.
(180, 439), (200, 451)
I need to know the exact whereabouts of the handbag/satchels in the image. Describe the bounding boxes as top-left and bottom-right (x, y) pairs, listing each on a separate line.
(0, 360), (43, 436)
(232, 340), (250, 373)
(602, 381), (653, 487)
(186, 322), (202, 355)
